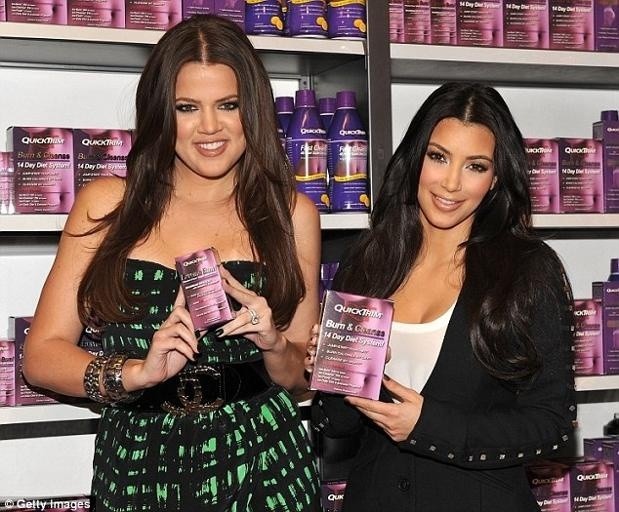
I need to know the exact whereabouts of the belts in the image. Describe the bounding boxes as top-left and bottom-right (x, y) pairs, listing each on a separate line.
(122, 354), (271, 414)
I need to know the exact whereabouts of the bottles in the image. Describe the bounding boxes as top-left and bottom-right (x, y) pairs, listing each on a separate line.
(317, 261), (343, 302)
(287, 90), (330, 214)
(318, 97), (335, 129)
(245, 0), (366, 42)
(275, 96), (294, 132)
(327, 91), (369, 215)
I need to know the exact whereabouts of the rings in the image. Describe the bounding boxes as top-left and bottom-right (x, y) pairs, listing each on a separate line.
(246, 308), (259, 325)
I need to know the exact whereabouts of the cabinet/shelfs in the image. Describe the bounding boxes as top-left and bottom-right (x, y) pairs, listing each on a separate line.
(0, 22), (373, 441)
(390, 42), (619, 404)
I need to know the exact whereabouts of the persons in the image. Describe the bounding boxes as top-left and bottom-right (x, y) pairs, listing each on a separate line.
(22, 15), (322, 512)
(305, 82), (577, 511)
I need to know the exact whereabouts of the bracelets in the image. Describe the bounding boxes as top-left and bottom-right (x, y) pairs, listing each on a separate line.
(84, 357), (113, 405)
(103, 355), (144, 403)
